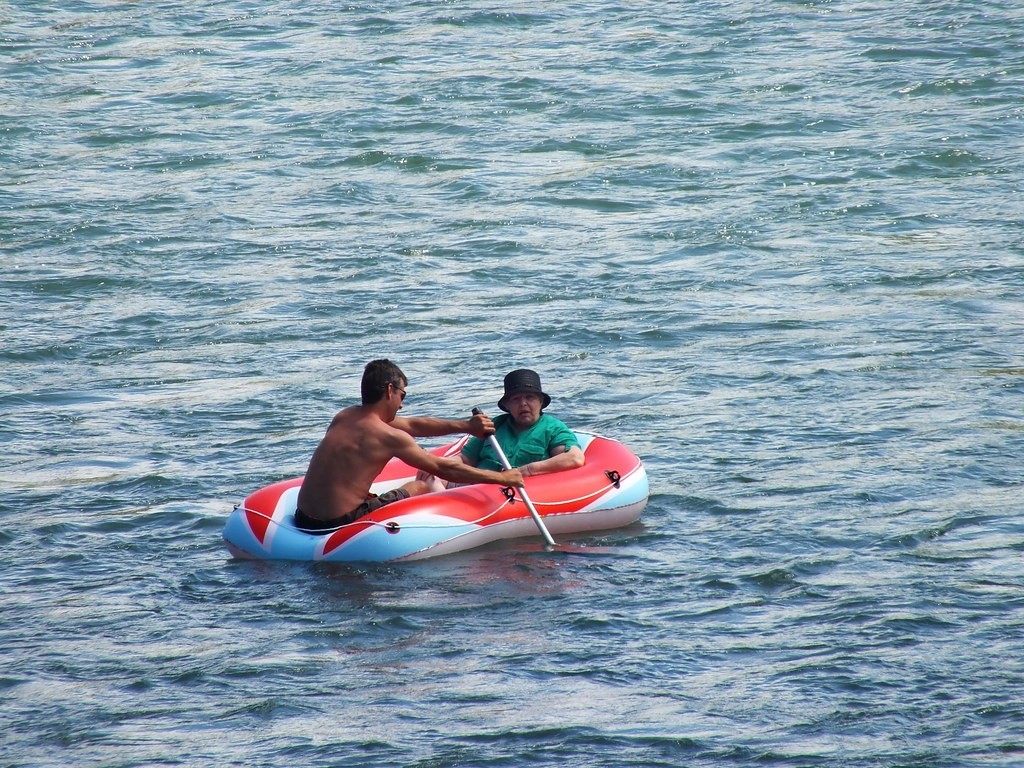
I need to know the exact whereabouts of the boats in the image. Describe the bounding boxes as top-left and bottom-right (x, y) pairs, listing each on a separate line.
(220, 427), (650, 565)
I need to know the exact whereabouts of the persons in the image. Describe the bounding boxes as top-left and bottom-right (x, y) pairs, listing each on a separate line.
(415, 368), (585, 489)
(294, 359), (525, 535)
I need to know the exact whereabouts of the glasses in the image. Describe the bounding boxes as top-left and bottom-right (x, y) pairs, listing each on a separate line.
(391, 384), (407, 400)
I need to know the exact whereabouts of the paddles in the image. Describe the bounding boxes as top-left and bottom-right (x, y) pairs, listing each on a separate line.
(471, 408), (562, 549)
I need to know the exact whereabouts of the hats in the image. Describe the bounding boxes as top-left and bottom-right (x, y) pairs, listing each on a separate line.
(497, 368), (551, 412)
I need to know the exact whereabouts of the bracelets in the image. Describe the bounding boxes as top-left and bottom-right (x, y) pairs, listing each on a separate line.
(526, 465), (533, 476)
